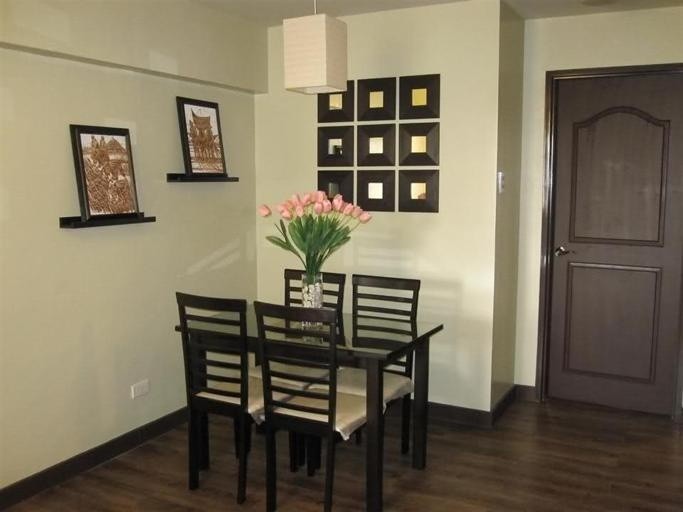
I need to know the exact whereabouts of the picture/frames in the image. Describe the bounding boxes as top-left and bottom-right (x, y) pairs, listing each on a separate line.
(67, 123), (141, 222)
(174, 96), (227, 177)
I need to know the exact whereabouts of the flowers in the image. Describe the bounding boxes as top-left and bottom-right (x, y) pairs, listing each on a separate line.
(254, 190), (374, 282)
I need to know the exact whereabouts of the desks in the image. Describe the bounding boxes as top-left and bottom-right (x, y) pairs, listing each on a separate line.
(175, 304), (444, 512)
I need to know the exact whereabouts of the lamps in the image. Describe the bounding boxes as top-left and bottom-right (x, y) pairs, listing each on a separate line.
(282, 1), (347, 96)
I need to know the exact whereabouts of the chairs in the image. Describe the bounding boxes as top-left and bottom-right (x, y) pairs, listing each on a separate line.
(174, 268), (421, 512)
